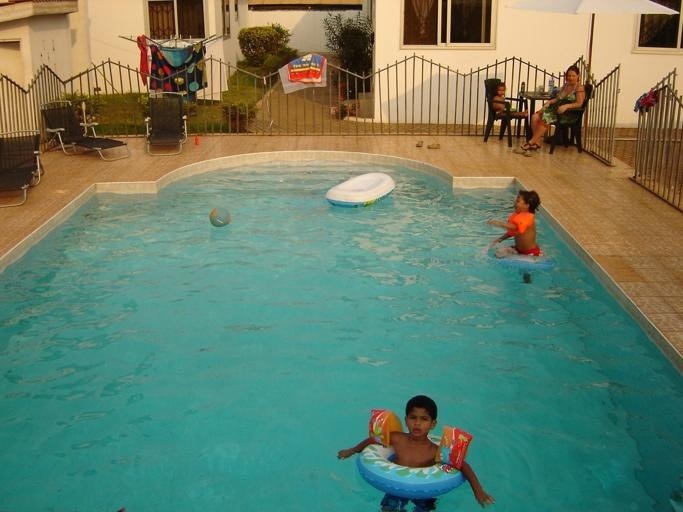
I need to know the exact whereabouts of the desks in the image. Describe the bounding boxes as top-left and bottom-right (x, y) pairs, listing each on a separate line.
(517, 91), (570, 142)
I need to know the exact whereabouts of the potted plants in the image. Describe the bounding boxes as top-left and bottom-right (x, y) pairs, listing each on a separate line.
(328, 70), (357, 101)
(253, 56), (284, 86)
(221, 101), (256, 129)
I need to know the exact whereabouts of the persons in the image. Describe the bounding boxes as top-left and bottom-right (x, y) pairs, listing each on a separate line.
(517, 63), (585, 151)
(491, 81), (527, 120)
(336, 393), (495, 512)
(483, 188), (544, 262)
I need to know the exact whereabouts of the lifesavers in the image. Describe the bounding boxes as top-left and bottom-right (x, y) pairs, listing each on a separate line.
(326, 171), (395, 208)
(356, 434), (467, 499)
(484, 249), (557, 271)
(369, 409), (472, 469)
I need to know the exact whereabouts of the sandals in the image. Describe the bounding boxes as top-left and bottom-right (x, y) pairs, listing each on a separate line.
(416, 140), (423, 147)
(429, 143), (439, 150)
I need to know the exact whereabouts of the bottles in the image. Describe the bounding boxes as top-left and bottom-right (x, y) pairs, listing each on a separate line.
(520, 82), (525, 93)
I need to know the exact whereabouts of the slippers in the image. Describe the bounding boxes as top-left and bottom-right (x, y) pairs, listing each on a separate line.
(513, 143), (542, 157)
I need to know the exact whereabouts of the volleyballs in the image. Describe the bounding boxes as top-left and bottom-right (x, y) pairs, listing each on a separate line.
(210, 207), (231, 227)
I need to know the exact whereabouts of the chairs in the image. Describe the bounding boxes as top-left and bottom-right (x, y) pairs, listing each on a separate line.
(482, 78), (528, 146)
(0, 100), (131, 208)
(545, 84), (593, 154)
(144, 91), (189, 156)
